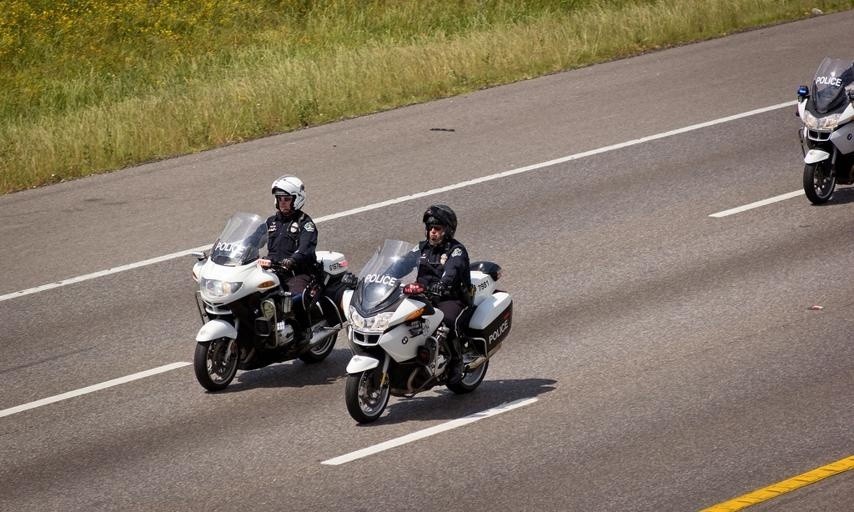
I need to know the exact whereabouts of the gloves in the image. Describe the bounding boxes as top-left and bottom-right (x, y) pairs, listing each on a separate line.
(430, 280), (447, 293)
(281, 256), (296, 268)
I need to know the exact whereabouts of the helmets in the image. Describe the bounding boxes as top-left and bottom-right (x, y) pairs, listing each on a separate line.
(422, 204), (458, 241)
(270, 173), (306, 214)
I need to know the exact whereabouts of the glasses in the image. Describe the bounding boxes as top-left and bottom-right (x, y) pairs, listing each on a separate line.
(276, 197), (293, 201)
(425, 225), (443, 231)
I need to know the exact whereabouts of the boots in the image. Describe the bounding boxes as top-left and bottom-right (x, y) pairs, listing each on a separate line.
(447, 335), (464, 384)
(292, 297), (313, 347)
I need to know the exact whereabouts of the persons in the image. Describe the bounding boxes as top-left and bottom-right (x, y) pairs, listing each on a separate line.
(830, 62), (854, 89)
(234, 174), (318, 358)
(379, 204), (476, 381)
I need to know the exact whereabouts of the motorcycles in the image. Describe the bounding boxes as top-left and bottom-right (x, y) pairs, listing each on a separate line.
(339, 237), (513, 423)
(192, 212), (348, 395)
(793, 54), (853, 205)
